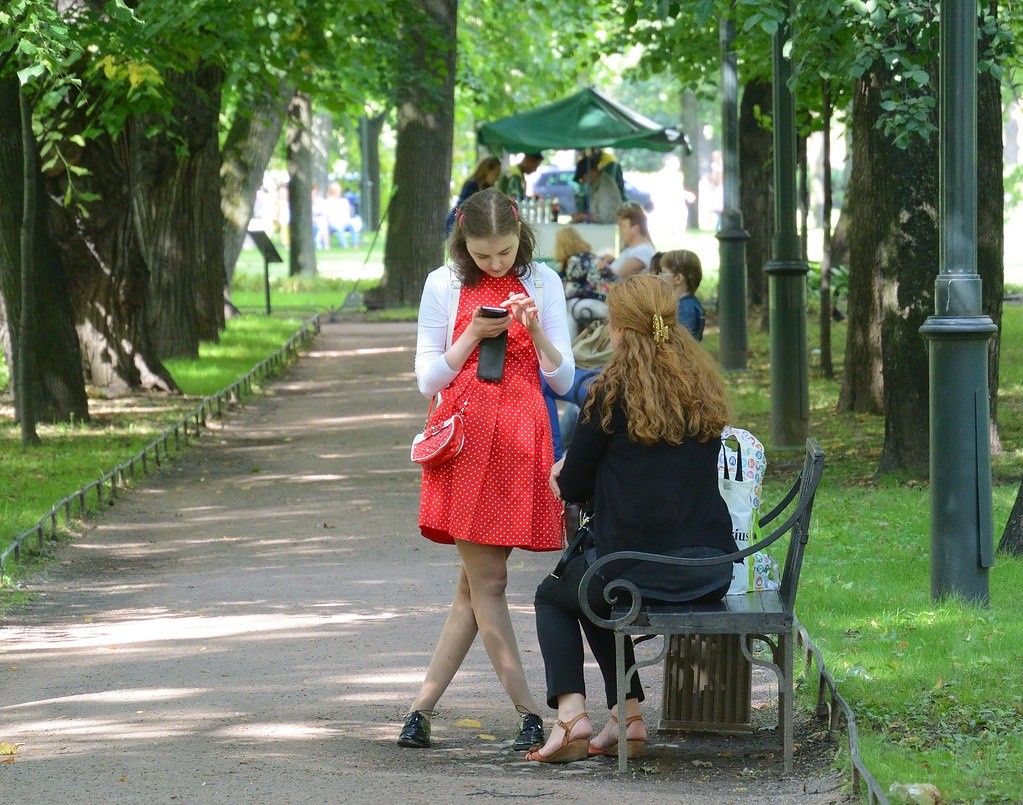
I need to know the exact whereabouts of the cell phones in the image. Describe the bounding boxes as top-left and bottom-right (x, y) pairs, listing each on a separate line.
(481, 306), (508, 319)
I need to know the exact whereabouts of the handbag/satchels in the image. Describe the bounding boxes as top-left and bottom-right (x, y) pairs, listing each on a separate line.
(552, 518), (598, 579)
(572, 318), (620, 369)
(412, 417), (468, 471)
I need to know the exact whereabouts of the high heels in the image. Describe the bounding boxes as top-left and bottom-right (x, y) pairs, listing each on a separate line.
(589, 711), (647, 761)
(526, 714), (593, 764)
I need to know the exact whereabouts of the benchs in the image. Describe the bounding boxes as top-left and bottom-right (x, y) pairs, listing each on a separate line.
(573, 296), (826, 773)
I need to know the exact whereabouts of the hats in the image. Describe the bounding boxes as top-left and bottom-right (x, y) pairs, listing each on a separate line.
(574, 159), (595, 182)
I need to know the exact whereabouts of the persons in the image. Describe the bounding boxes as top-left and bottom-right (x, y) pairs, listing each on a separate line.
(524, 273), (744, 763)
(572, 158), (621, 224)
(311, 181), (359, 251)
(552, 227), (618, 340)
(497, 153), (543, 201)
(446, 157), (502, 238)
(609, 207), (655, 279)
(653, 155), (696, 247)
(583, 148), (626, 200)
(278, 181), (289, 248)
(649, 250), (706, 342)
(396, 187), (576, 749)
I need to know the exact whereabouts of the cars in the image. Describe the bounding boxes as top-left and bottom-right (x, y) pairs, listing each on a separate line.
(533, 167), (656, 221)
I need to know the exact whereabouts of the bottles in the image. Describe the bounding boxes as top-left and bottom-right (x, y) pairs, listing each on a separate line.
(514, 195), (559, 223)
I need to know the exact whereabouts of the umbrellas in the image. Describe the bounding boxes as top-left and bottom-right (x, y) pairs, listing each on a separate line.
(478, 87), (694, 156)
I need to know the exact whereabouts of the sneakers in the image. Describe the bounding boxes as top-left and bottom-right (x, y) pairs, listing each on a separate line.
(398, 709), (433, 748)
(512, 705), (544, 751)
